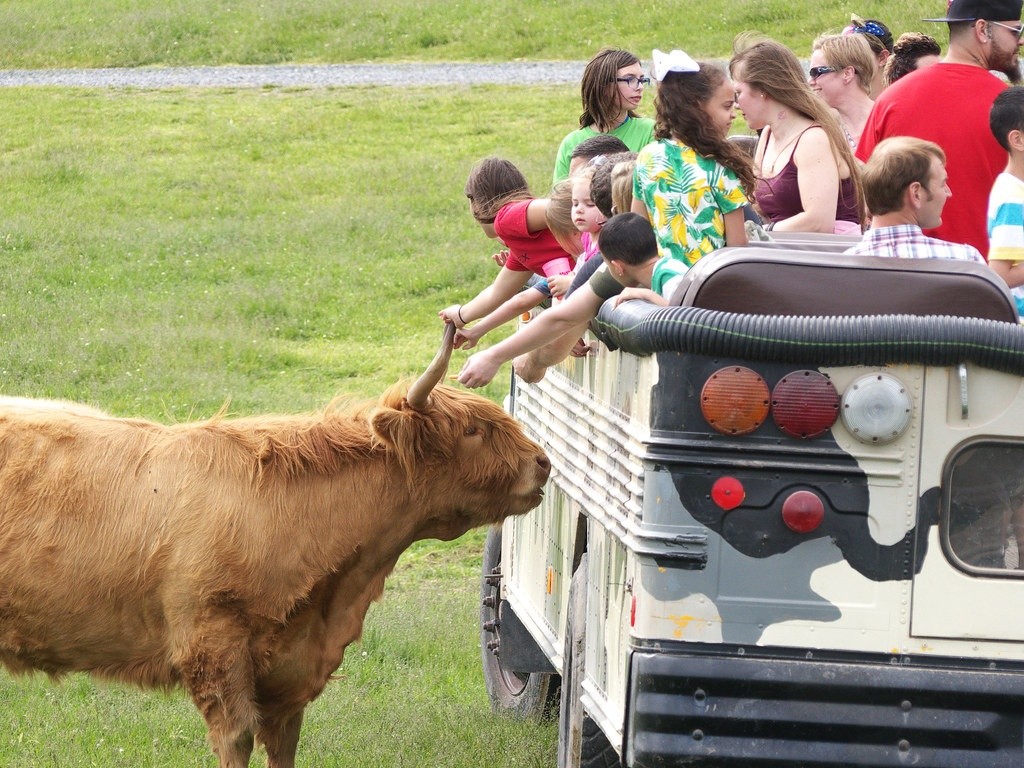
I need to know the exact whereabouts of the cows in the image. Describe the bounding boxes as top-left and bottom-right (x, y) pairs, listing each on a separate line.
(0, 320), (551, 768)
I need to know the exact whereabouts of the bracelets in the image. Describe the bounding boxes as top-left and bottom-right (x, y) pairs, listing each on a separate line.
(459, 305), (468, 325)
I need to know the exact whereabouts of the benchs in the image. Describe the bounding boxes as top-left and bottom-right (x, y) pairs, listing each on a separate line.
(667, 247), (1020, 324)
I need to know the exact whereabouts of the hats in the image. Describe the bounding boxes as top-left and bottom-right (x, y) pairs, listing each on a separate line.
(922, 0), (1023, 23)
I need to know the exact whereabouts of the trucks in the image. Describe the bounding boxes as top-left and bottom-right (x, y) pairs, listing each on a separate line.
(476, 234), (1024, 768)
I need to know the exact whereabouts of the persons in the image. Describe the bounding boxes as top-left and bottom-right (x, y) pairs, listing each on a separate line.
(597, 212), (690, 310)
(841, 134), (987, 267)
(808, 31), (876, 143)
(628, 47), (776, 271)
(438, 0), (1024, 391)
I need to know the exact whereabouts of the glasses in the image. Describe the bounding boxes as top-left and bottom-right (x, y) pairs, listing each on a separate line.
(810, 65), (859, 80)
(610, 76), (651, 89)
(596, 212), (609, 227)
(971, 21), (1024, 40)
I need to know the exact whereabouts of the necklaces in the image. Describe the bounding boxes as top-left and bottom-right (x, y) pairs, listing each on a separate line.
(771, 120), (814, 172)
